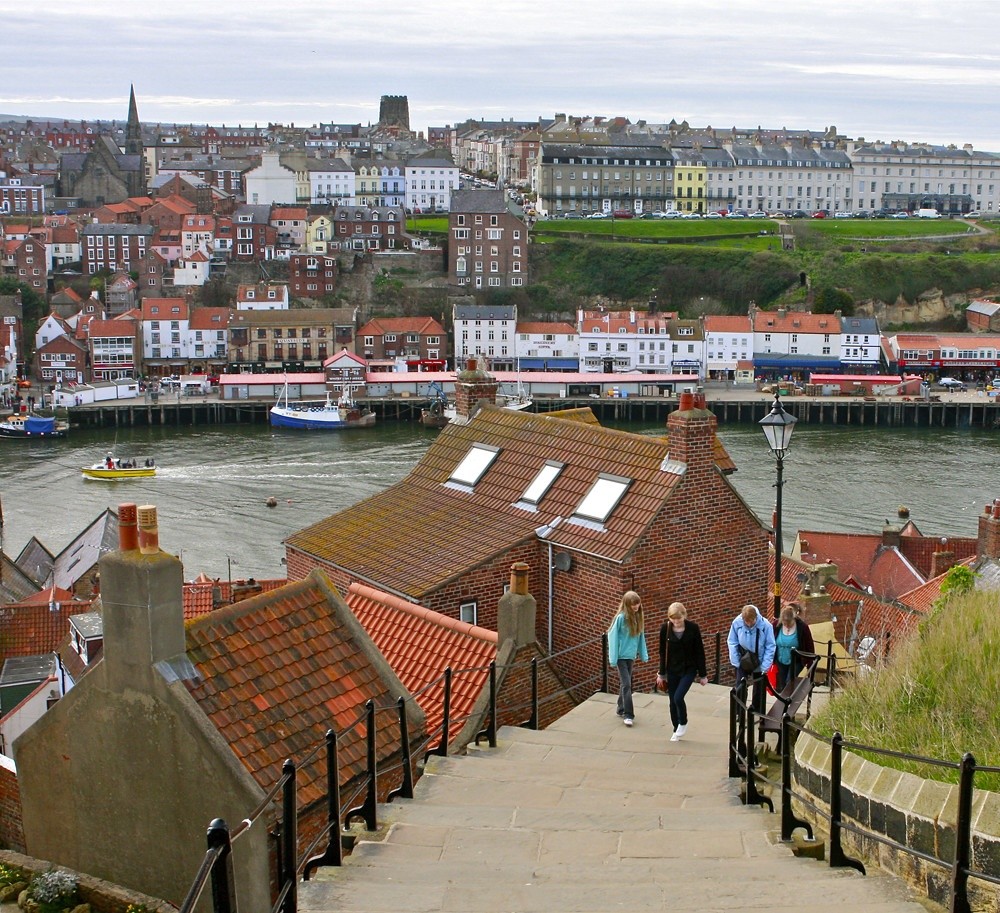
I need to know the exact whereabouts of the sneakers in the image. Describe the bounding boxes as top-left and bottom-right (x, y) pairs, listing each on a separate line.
(670, 732), (680, 742)
(676, 722), (688, 737)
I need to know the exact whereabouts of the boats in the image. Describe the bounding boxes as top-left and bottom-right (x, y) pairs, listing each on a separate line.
(0, 410), (71, 440)
(80, 432), (156, 479)
(268, 369), (377, 430)
(420, 352), (534, 429)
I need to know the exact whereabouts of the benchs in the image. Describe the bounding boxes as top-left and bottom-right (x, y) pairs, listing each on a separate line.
(758, 648), (820, 751)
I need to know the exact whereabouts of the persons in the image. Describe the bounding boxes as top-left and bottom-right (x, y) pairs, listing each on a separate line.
(106, 456), (154, 469)
(75, 394), (83, 406)
(772, 601), (815, 694)
(657, 602), (708, 741)
(605, 591), (649, 725)
(727, 605), (776, 723)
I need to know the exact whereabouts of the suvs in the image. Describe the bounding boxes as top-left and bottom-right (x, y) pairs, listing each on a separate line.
(938, 378), (964, 388)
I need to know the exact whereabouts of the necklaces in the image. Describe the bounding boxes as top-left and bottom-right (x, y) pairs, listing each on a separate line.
(784, 622), (795, 632)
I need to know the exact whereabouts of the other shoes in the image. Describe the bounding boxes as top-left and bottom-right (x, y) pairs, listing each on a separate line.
(616, 711), (626, 719)
(623, 718), (633, 726)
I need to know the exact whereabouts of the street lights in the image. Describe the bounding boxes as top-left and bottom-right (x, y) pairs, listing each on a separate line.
(758, 384), (801, 665)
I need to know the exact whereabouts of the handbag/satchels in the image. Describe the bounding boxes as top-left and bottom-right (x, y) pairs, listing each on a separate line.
(739, 651), (760, 672)
(766, 664), (777, 697)
(659, 679), (668, 692)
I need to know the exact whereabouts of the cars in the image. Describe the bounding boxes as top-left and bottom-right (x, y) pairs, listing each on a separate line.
(15, 377), (32, 388)
(992, 378), (1000, 387)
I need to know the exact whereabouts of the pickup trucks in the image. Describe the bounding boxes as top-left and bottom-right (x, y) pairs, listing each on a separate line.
(159, 376), (179, 388)
